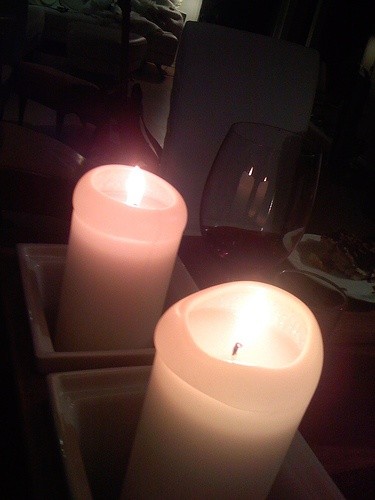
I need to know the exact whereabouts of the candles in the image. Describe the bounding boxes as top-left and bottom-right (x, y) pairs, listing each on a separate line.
(231, 166), (270, 227)
(58, 164), (188, 352)
(126, 281), (323, 500)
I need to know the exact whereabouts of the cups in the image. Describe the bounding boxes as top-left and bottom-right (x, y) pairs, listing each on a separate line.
(199, 122), (321, 281)
(276, 270), (348, 339)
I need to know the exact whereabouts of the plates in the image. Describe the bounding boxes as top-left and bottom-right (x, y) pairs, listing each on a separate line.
(282, 228), (375, 303)
